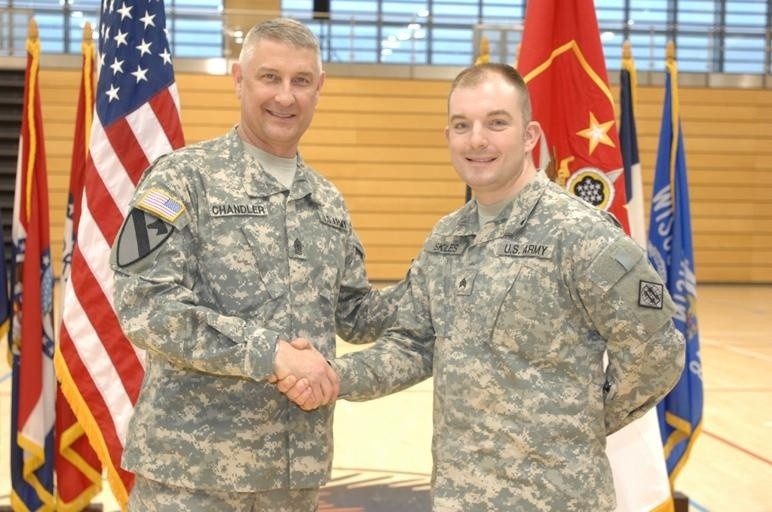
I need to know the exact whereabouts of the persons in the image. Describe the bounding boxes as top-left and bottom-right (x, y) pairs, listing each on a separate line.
(106, 16), (412, 511)
(267, 62), (689, 512)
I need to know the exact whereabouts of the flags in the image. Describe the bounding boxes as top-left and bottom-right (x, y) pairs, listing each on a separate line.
(51, 45), (104, 511)
(51, 2), (186, 510)
(466, 1), (705, 512)
(11, 39), (52, 510)
